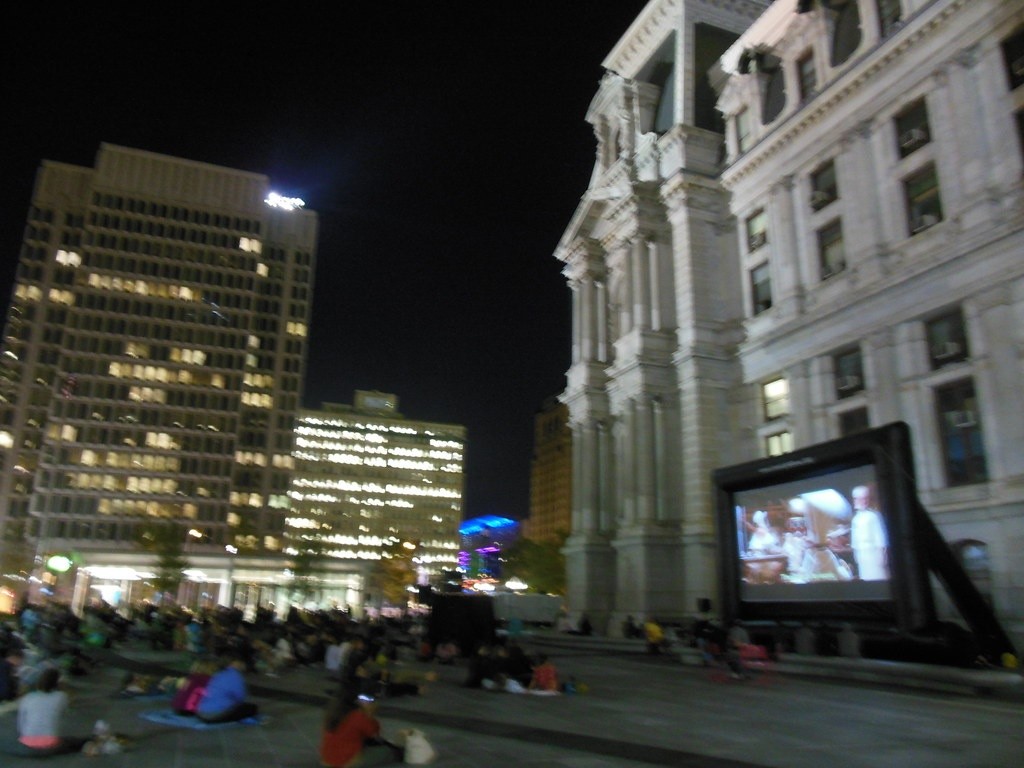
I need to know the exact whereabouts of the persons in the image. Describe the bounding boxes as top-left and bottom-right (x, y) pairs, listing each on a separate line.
(1, 591), (863, 767)
(748, 486), (888, 584)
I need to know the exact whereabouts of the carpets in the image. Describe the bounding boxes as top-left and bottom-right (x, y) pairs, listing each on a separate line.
(137, 706), (271, 730)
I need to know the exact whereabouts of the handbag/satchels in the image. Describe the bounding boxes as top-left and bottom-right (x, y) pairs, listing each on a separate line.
(399, 727), (437, 765)
(562, 680), (577, 693)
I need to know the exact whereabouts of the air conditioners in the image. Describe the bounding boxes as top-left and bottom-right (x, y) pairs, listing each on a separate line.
(951, 410), (978, 429)
(898, 128), (921, 148)
(834, 373), (861, 391)
(930, 341), (963, 361)
(748, 232), (765, 248)
(806, 189), (824, 207)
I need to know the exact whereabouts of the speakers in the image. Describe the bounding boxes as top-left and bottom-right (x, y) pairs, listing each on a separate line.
(697, 598), (711, 613)
